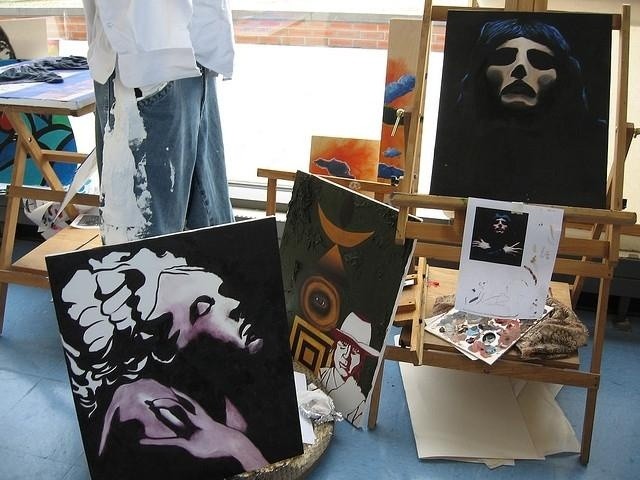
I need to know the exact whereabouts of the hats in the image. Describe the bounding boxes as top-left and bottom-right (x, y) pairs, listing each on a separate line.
(335, 311), (380, 357)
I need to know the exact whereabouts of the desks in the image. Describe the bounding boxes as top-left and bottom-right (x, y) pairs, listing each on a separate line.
(0, 60), (110, 330)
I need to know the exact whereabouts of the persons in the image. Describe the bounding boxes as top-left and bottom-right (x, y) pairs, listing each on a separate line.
(456, 18), (602, 208)
(473, 210), (522, 266)
(82, 1), (236, 247)
(58, 249), (271, 479)
(316, 312), (382, 428)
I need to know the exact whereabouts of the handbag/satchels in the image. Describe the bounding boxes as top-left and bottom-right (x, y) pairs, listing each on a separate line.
(432, 293), (590, 361)
